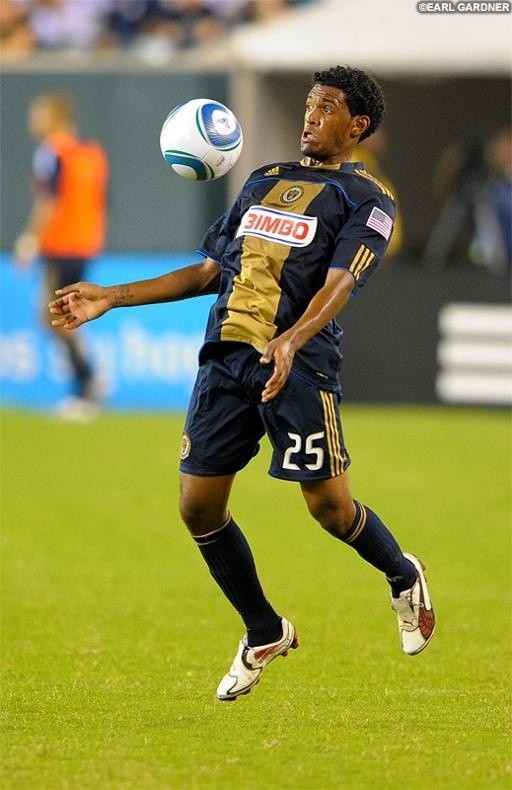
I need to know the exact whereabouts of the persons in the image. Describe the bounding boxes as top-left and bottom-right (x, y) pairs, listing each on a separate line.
(42, 63), (434, 705)
(11, 83), (113, 423)
(351, 135), (408, 259)
(1, 1), (330, 63)
(457, 133), (512, 277)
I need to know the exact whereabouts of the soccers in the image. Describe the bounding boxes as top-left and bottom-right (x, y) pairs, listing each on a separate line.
(160, 98), (243, 182)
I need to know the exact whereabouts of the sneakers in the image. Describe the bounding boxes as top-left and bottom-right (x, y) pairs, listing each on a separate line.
(390, 552), (437, 656)
(216, 615), (299, 701)
